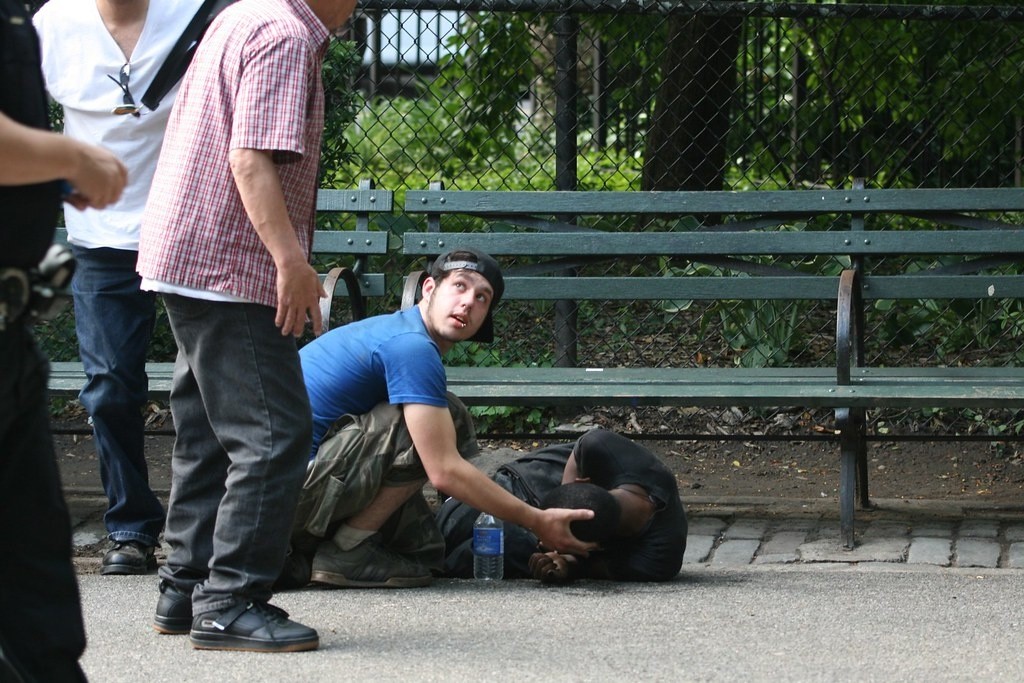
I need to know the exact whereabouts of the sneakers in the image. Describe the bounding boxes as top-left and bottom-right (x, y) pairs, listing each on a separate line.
(310, 538), (433, 588)
(189, 600), (320, 652)
(152, 578), (192, 634)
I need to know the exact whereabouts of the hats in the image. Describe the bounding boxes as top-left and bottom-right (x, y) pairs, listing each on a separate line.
(433, 247), (504, 343)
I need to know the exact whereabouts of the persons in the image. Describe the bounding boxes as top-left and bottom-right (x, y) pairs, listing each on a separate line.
(298, 247), (688, 584)
(0, 0), (128, 683)
(31, 0), (218, 575)
(152, 0), (358, 652)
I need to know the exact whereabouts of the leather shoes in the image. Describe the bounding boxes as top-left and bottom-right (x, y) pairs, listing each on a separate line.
(99, 540), (156, 573)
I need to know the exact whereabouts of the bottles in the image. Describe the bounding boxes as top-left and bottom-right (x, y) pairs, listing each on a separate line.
(473, 512), (504, 581)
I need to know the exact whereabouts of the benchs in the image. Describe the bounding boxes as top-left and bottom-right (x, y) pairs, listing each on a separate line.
(403, 179), (1024, 553)
(0, 178), (395, 502)
